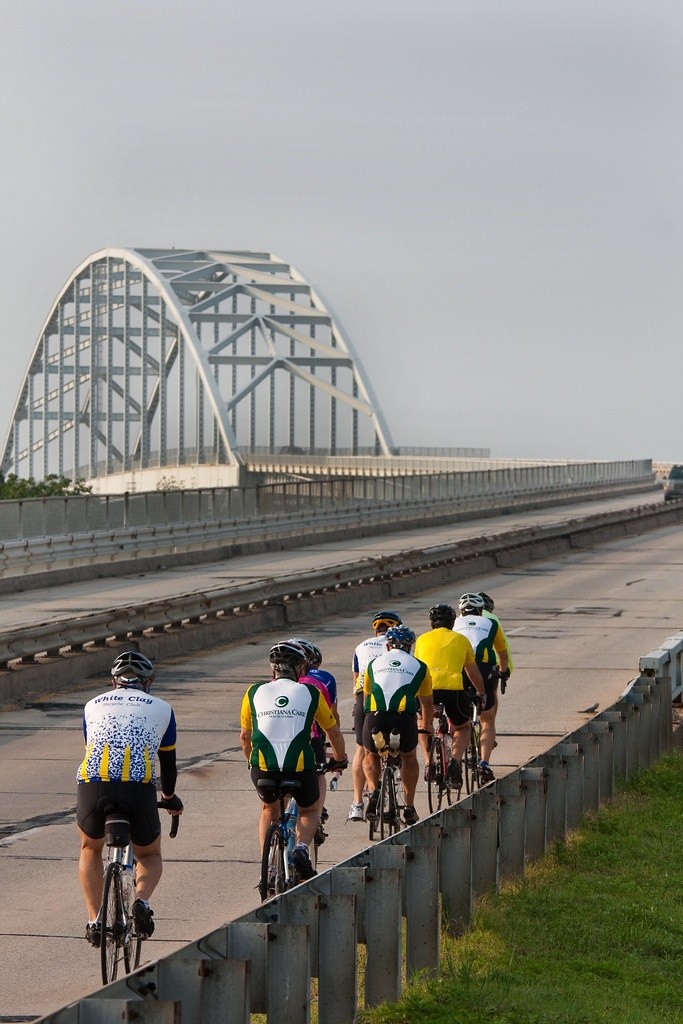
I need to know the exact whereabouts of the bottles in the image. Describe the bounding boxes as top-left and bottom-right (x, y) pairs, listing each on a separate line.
(122, 865), (128, 891)
(125, 863), (133, 893)
(444, 733), (451, 762)
(390, 729), (400, 757)
(371, 727), (388, 756)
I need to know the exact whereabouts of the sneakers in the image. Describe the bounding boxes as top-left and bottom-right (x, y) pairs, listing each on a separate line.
(130, 900), (155, 941)
(402, 806), (418, 821)
(444, 763), (464, 791)
(363, 791), (382, 819)
(292, 846), (316, 877)
(84, 924), (103, 942)
(348, 803), (366, 820)
(424, 763), (437, 781)
(474, 763), (497, 782)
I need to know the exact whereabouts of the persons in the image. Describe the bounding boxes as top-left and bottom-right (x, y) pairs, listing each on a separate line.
(349, 591), (513, 825)
(76, 651), (184, 949)
(241, 638), (348, 901)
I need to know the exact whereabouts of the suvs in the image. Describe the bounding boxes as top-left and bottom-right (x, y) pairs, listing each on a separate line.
(663, 465), (683, 501)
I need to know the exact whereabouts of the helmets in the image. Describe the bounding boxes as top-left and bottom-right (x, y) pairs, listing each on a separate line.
(477, 589), (497, 610)
(308, 643), (323, 665)
(429, 603), (458, 623)
(295, 639), (316, 662)
(458, 591), (483, 612)
(384, 625), (417, 645)
(269, 639), (310, 667)
(373, 609), (404, 630)
(111, 651), (156, 680)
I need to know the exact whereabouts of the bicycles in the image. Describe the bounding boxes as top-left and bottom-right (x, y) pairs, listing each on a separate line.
(363, 711), (497, 840)
(247, 756), (347, 903)
(85, 800), (180, 986)
